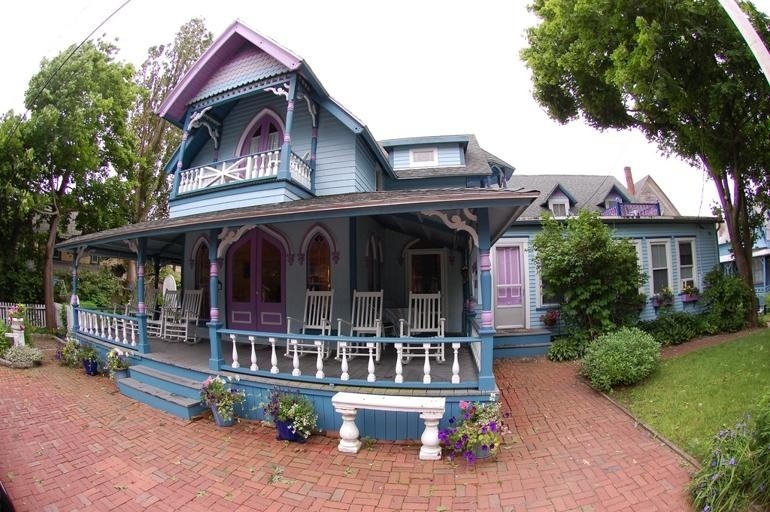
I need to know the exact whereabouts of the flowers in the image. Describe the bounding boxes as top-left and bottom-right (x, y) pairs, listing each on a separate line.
(7, 301), (25, 329)
(541, 309), (561, 322)
(654, 284), (701, 300)
(200, 373), (321, 438)
(437, 393), (512, 465)
(62, 337), (134, 368)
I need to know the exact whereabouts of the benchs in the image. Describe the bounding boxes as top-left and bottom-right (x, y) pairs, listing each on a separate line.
(330, 391), (447, 461)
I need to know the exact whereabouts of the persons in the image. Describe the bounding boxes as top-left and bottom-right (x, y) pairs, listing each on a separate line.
(262, 281), (274, 303)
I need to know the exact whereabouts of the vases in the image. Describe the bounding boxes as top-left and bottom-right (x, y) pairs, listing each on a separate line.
(472, 439), (494, 459)
(83, 360), (128, 389)
(276, 420), (304, 442)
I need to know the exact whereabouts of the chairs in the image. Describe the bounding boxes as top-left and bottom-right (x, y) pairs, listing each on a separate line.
(111, 286), (204, 345)
(284, 287), (447, 364)
